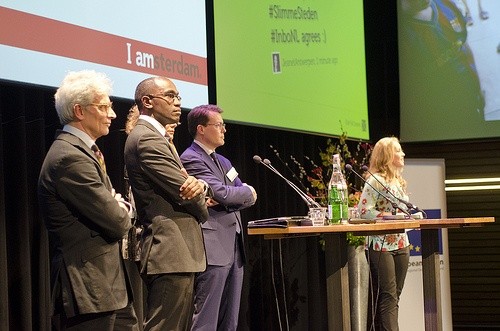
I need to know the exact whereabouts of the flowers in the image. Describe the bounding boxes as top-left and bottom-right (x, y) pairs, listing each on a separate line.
(269, 126), (376, 249)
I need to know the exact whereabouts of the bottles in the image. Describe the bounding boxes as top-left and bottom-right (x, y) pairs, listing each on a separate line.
(328, 158), (343, 225)
(333, 154), (349, 223)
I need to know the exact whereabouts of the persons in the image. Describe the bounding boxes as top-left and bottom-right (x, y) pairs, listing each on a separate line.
(120, 103), (181, 331)
(397, 0), (485, 141)
(38, 70), (139, 331)
(357, 136), (423, 331)
(125, 77), (214, 331)
(179, 105), (257, 331)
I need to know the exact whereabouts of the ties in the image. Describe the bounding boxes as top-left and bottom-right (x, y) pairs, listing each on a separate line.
(92, 145), (106, 176)
(165, 132), (180, 161)
(210, 152), (223, 174)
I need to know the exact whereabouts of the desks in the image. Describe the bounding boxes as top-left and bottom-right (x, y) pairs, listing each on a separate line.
(247, 217), (495, 331)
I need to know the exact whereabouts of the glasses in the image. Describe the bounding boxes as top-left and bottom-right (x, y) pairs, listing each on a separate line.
(80, 101), (113, 113)
(145, 93), (181, 105)
(201, 122), (226, 129)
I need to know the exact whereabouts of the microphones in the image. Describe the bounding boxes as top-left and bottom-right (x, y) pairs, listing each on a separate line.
(264, 159), (329, 220)
(253, 155), (314, 219)
(345, 164), (414, 220)
(362, 166), (427, 219)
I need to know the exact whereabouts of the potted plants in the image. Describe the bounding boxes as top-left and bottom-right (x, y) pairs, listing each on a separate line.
(319, 233), (369, 331)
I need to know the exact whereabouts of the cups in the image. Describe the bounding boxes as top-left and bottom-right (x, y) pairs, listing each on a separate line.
(310, 207), (327, 226)
